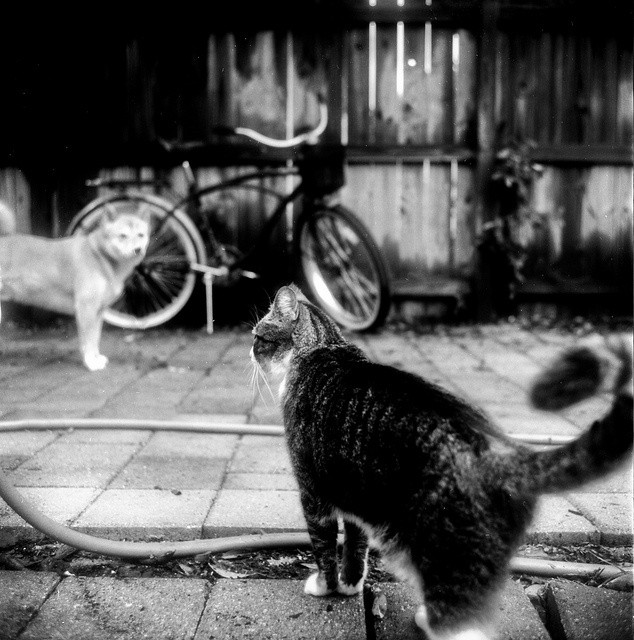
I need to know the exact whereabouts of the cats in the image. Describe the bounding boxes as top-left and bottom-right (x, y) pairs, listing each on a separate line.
(247, 278), (633, 640)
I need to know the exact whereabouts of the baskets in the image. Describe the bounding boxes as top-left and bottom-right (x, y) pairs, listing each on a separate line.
(296, 143), (346, 194)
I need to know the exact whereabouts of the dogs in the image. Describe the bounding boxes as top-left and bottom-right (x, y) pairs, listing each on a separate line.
(0, 198), (154, 371)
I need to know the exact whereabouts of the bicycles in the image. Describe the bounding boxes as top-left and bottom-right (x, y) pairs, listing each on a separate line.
(65, 91), (391, 336)
